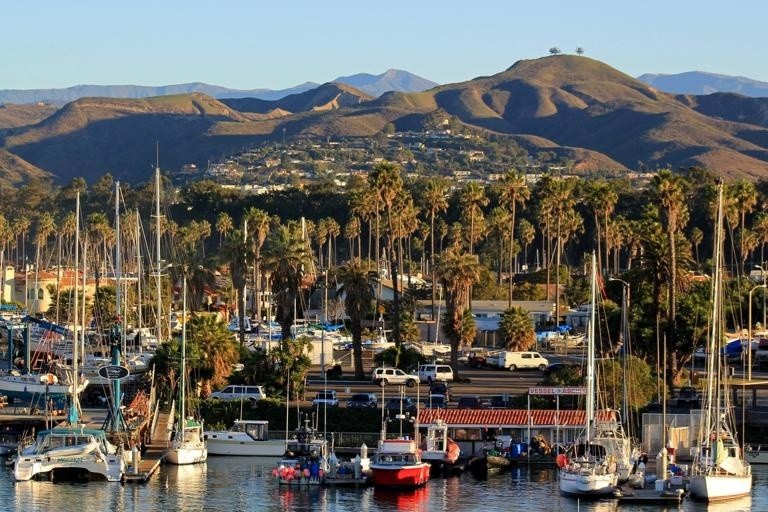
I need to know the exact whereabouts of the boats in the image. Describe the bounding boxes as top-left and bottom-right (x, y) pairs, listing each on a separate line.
(202, 419), (287, 456)
(277, 413), (462, 487)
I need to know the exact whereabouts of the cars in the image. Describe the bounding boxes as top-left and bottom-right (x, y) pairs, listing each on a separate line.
(430, 381), (451, 394)
(312, 390), (340, 406)
(457, 393), (482, 410)
(385, 395), (415, 411)
(346, 392), (378, 408)
(370, 367), (420, 388)
(424, 393), (448, 409)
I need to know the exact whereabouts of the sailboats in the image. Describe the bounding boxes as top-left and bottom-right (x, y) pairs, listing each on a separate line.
(168, 273), (207, 465)
(558, 249), (647, 500)
(687, 176), (754, 501)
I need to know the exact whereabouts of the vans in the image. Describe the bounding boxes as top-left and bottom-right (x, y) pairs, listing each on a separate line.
(211, 384), (266, 399)
(499, 349), (548, 372)
(409, 363), (454, 381)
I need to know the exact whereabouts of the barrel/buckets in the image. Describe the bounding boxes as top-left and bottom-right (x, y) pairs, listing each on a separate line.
(310, 465), (319, 476)
(511, 443), (521, 457)
(521, 442), (529, 452)
